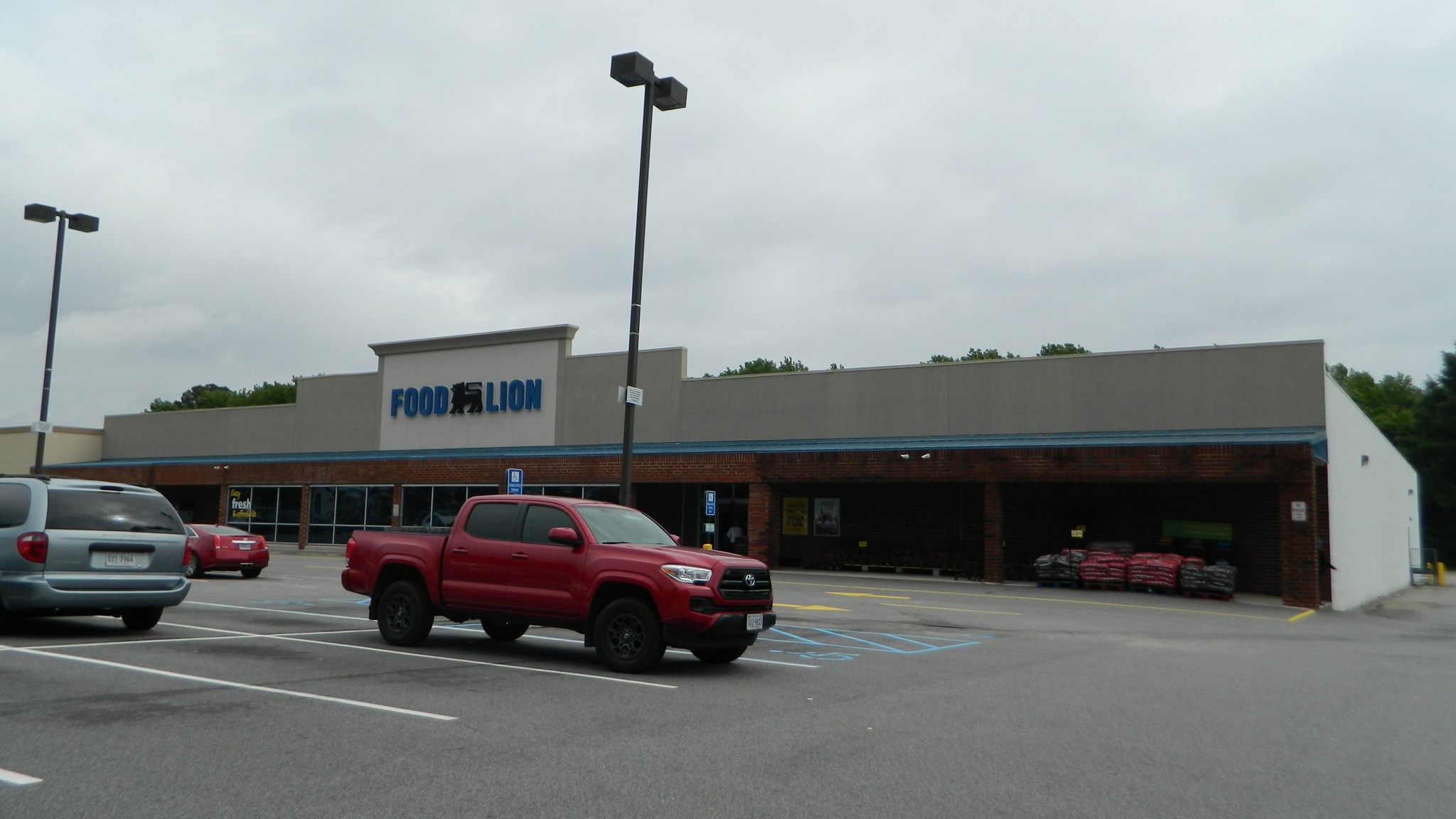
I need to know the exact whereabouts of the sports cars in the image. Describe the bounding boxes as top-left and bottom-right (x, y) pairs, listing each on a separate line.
(183, 523), (270, 580)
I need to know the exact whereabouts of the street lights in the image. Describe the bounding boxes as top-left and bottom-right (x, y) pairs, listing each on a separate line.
(22, 203), (100, 473)
(611, 51), (687, 507)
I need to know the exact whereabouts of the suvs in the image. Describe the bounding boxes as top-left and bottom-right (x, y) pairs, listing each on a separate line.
(0, 473), (193, 638)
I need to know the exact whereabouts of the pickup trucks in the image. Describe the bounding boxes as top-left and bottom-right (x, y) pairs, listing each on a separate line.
(342, 494), (777, 676)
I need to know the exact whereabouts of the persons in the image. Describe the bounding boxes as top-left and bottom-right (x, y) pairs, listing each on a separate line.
(726, 520), (742, 542)
(815, 513), (838, 536)
